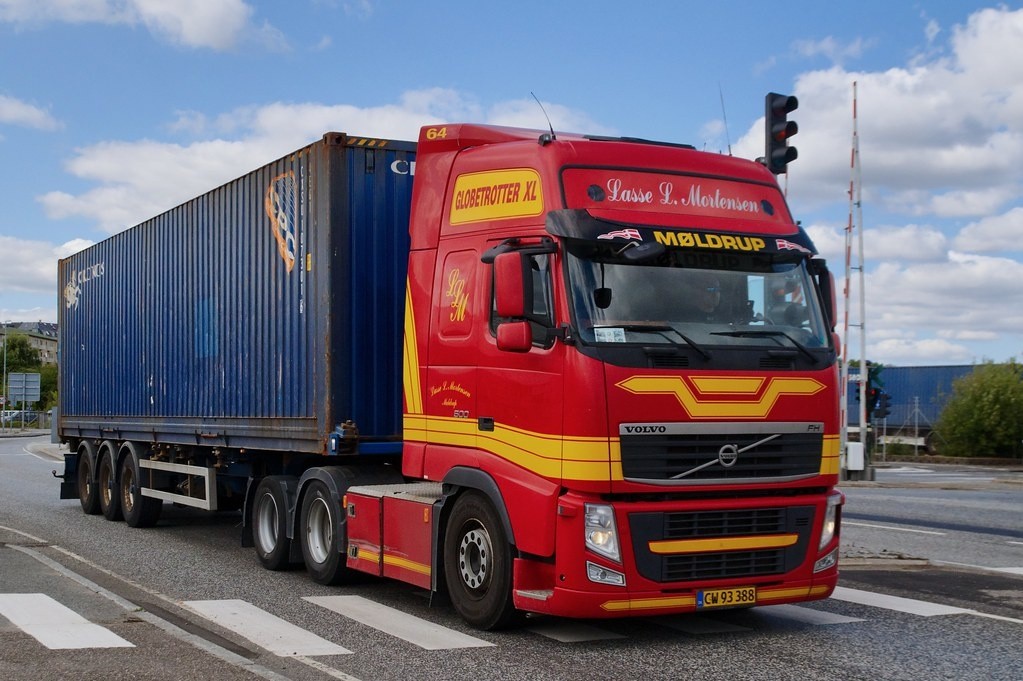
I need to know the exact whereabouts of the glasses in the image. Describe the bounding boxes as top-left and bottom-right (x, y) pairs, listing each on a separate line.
(692, 286), (722, 293)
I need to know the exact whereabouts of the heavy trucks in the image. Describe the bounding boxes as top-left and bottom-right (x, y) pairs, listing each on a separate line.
(52, 124), (848, 633)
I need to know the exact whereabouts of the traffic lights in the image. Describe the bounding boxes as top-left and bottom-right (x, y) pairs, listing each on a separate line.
(879, 393), (893, 418)
(864, 388), (879, 412)
(763, 92), (799, 176)
(856, 381), (869, 401)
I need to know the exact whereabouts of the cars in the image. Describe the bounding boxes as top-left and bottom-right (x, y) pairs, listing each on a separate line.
(0, 410), (39, 424)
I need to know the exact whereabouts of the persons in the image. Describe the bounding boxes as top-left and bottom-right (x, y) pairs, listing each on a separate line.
(681, 272), (737, 323)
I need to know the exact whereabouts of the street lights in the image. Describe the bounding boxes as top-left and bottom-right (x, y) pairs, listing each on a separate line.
(1, 320), (13, 434)
